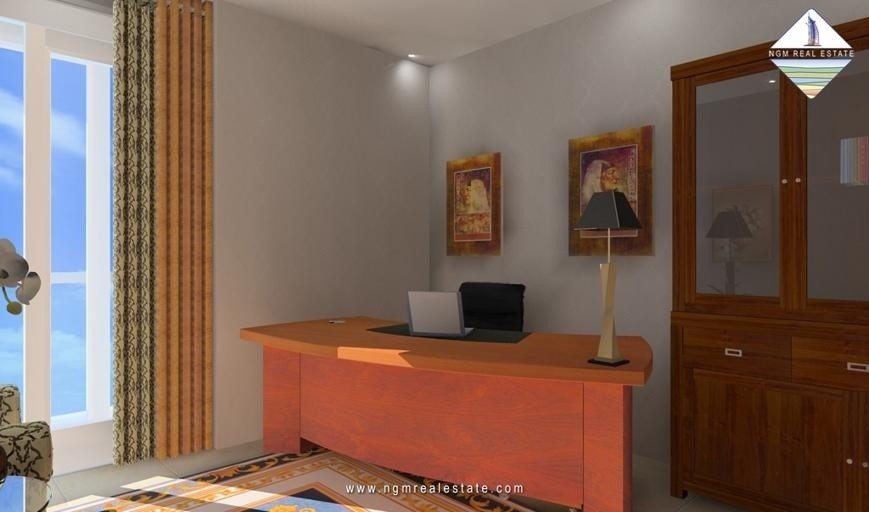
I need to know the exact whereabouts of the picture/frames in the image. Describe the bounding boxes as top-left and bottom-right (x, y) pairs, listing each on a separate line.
(567, 124), (655, 256)
(446, 154), (504, 257)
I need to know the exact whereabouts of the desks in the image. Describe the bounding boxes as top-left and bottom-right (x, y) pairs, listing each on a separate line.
(238, 315), (654, 512)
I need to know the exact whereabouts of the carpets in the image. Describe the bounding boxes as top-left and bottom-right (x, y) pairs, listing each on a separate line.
(52, 442), (584, 512)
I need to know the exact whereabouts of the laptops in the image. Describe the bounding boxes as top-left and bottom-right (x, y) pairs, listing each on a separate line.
(407, 291), (475, 338)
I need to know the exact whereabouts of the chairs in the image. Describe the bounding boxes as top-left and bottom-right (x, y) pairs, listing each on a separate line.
(459, 281), (526, 331)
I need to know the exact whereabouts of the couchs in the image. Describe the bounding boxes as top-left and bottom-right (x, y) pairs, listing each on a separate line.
(0, 385), (53, 512)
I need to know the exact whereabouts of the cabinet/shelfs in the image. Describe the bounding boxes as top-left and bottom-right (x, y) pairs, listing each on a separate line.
(677, 60), (793, 303)
(670, 309), (864, 511)
(793, 45), (864, 305)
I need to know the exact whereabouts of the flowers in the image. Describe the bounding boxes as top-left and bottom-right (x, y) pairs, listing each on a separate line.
(0, 238), (42, 315)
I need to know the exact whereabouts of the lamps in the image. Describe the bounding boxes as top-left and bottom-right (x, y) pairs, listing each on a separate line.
(573, 191), (643, 367)
